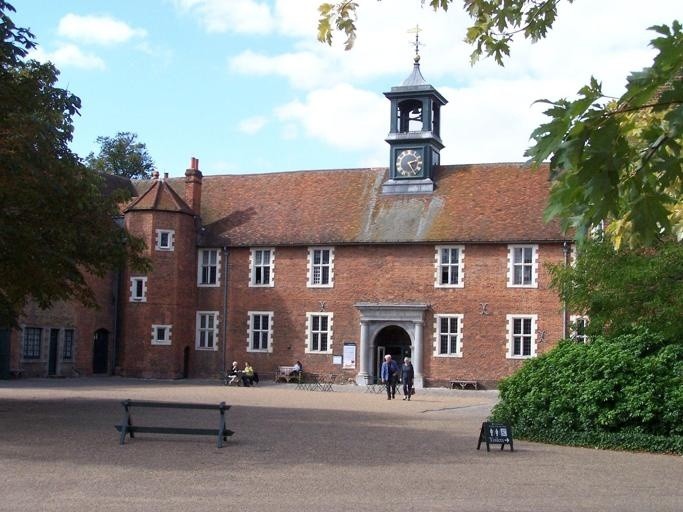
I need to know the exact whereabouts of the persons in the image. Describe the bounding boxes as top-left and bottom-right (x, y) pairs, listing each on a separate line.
(288, 361), (301, 375)
(398, 356), (415, 401)
(226, 361), (241, 385)
(380, 354), (400, 400)
(238, 361), (254, 387)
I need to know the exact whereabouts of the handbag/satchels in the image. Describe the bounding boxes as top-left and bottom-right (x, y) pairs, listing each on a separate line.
(411, 387), (415, 395)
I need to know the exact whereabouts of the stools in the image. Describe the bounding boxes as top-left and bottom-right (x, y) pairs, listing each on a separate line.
(295, 373), (338, 393)
(364, 376), (402, 394)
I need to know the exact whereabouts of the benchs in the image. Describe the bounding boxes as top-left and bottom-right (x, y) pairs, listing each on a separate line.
(275, 365), (302, 384)
(450, 380), (478, 391)
(224, 367), (253, 386)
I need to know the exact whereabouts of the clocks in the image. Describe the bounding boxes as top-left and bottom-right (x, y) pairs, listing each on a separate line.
(396, 150), (422, 177)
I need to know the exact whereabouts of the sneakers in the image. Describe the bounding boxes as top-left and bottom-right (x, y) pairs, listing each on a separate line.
(402, 397), (411, 401)
(387, 395), (395, 400)
